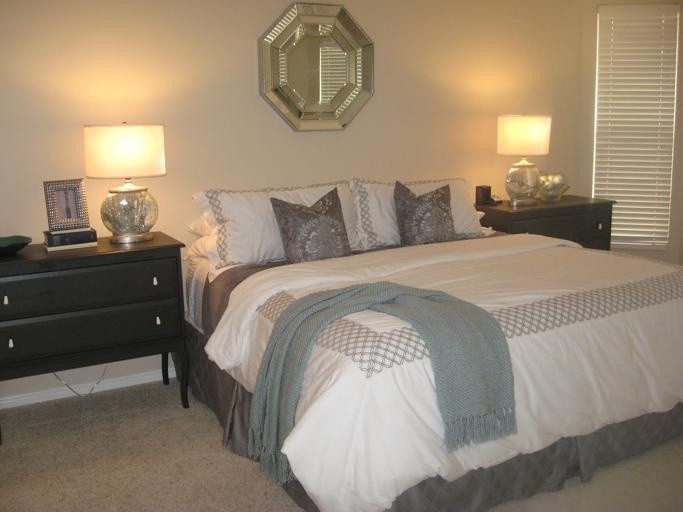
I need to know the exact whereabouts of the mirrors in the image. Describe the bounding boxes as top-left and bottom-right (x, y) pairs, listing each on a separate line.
(258, 2), (374, 132)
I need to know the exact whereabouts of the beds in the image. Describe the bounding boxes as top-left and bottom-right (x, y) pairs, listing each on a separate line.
(183, 228), (683, 512)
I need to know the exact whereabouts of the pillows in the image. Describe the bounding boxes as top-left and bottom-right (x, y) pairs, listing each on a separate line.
(270, 188), (351, 264)
(351, 174), (495, 251)
(394, 181), (457, 246)
(193, 180), (365, 284)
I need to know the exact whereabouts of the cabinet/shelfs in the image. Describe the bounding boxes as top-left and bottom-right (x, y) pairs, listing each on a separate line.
(1, 231), (191, 448)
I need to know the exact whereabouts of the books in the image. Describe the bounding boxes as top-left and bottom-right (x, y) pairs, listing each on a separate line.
(43, 227), (97, 252)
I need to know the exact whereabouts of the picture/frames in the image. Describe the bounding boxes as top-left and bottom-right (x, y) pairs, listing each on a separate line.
(44, 179), (91, 230)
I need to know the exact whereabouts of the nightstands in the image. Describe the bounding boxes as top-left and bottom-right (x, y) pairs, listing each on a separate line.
(474, 194), (618, 251)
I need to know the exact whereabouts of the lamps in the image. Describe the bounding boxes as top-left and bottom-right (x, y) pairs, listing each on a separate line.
(497, 114), (552, 207)
(83, 121), (167, 245)
